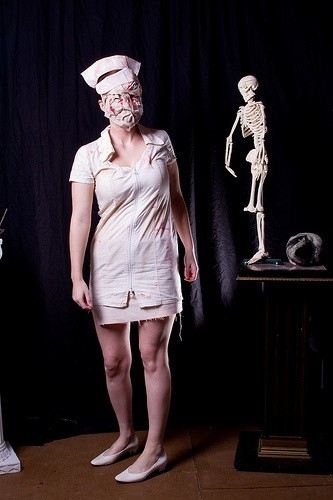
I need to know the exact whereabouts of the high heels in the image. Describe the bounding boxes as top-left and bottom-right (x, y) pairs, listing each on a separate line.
(115, 449), (167, 482)
(91, 437), (138, 465)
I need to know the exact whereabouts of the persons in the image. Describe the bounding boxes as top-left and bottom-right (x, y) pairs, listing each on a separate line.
(67, 56), (202, 484)
